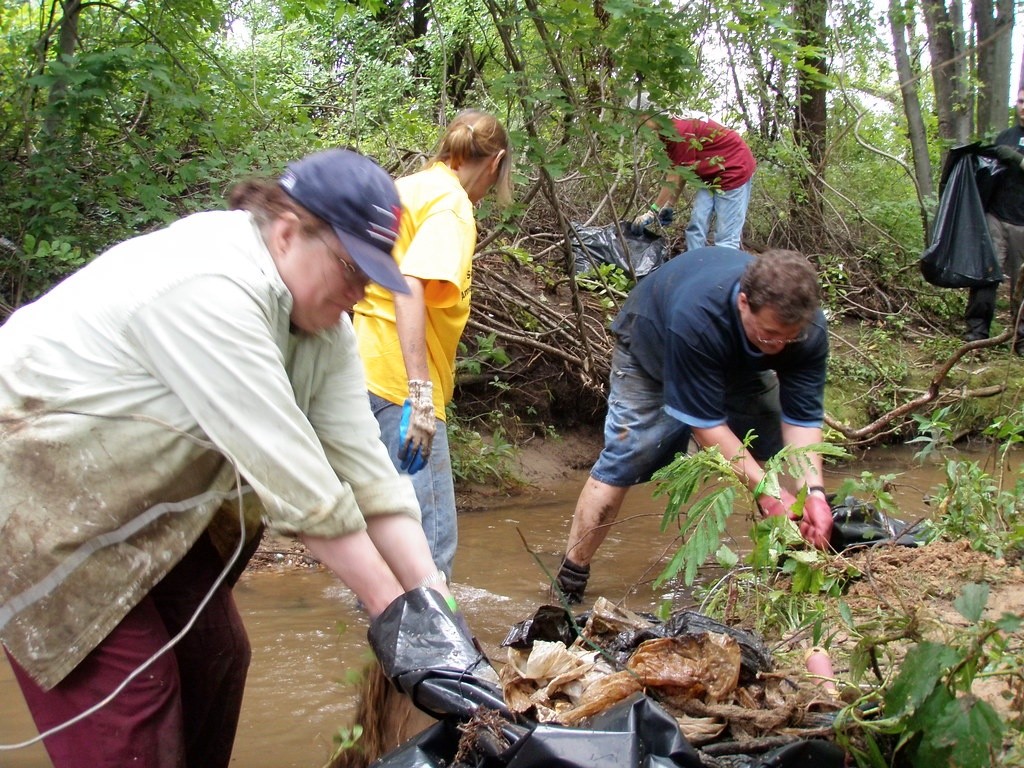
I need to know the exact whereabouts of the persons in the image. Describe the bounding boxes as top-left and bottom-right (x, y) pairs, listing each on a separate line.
(0, 147), (512, 768)
(549, 247), (829, 607)
(629, 92), (757, 254)
(351, 109), (515, 620)
(961, 86), (1024, 358)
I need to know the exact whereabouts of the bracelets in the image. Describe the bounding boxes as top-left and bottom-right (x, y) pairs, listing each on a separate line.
(412, 569), (447, 591)
(810, 486), (826, 494)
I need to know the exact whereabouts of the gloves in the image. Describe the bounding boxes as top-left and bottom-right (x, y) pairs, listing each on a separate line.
(788, 495), (833, 555)
(657, 201), (674, 225)
(993, 144), (1024, 166)
(759, 487), (802, 549)
(398, 380), (437, 475)
(631, 210), (655, 235)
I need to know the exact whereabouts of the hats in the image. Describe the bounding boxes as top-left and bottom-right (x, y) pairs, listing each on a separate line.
(629, 92), (668, 115)
(278, 148), (413, 295)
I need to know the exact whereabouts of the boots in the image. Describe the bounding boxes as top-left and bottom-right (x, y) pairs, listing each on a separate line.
(551, 554), (590, 607)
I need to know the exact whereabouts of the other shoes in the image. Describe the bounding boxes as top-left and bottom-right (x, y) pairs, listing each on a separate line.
(960, 332), (987, 341)
(1015, 346), (1024, 357)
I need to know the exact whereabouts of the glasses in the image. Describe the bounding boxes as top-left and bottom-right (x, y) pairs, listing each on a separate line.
(747, 301), (808, 346)
(277, 200), (372, 302)
